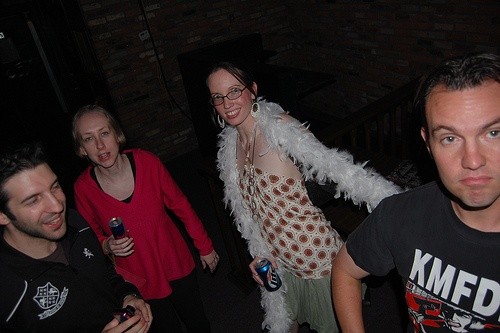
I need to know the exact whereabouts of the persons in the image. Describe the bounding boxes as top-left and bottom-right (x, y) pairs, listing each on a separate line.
(331, 51), (500, 333)
(72, 106), (219, 333)
(0, 146), (153, 333)
(206, 62), (410, 333)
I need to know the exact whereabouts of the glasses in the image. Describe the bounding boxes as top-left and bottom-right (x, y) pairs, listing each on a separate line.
(210, 86), (246, 106)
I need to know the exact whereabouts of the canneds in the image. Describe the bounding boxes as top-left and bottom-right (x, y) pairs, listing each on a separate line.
(255, 258), (282, 292)
(108, 216), (129, 240)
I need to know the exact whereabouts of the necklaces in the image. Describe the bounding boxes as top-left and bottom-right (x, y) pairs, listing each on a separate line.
(236, 126), (257, 222)
